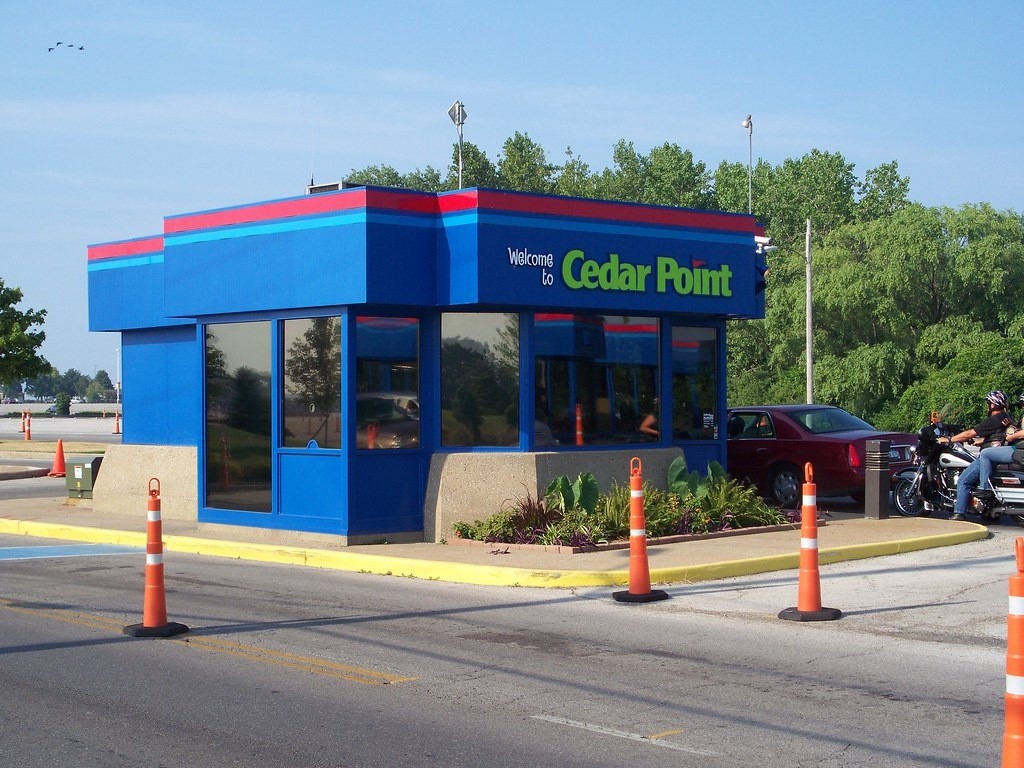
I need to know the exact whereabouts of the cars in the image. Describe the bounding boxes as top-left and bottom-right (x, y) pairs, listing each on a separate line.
(71, 398), (84, 403)
(46, 405), (56, 413)
(358, 392), (418, 420)
(358, 398), (420, 448)
(1, 397), (23, 404)
(44, 396), (56, 403)
(728, 405), (922, 511)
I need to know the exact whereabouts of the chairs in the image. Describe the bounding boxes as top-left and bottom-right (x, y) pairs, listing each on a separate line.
(728, 416), (746, 437)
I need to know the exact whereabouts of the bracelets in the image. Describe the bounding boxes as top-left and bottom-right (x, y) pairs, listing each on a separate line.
(948, 437), (951, 443)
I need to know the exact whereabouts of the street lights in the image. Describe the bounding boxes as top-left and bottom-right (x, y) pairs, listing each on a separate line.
(764, 245), (813, 430)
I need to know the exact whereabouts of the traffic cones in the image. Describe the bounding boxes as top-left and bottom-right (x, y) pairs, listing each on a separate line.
(47, 439), (67, 477)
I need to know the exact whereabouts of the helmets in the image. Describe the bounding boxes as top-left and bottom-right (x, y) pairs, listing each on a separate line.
(1019, 392), (1024, 402)
(985, 392), (1007, 408)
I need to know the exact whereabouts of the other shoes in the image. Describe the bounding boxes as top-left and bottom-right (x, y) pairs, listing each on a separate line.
(970, 489), (994, 497)
(950, 513), (965, 521)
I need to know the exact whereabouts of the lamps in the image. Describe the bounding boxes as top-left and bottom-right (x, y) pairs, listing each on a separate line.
(742, 114), (752, 214)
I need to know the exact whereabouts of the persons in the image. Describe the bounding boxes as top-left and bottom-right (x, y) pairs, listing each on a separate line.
(757, 416), (767, 426)
(970, 393), (1024, 494)
(640, 396), (659, 436)
(535, 409), (552, 445)
(940, 390), (1016, 521)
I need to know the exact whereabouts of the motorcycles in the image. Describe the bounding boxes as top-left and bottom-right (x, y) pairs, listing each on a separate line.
(892, 420), (1024, 525)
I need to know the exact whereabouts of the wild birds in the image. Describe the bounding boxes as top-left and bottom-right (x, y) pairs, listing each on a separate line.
(48, 48), (54, 52)
(56, 42), (62, 46)
(68, 44), (73, 47)
(78, 46), (83, 50)
